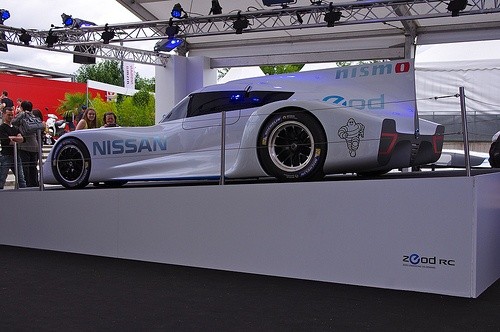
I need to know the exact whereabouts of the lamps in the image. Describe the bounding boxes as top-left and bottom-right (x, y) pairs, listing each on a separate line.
(323, 2), (342, 28)
(446, 0), (468, 17)
(171, 3), (188, 19)
(153, 37), (184, 51)
(233, 10), (254, 34)
(208, 0), (222, 15)
(0, 9), (116, 48)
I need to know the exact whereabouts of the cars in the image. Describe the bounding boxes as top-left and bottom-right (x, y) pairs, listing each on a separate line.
(489, 129), (500, 172)
(388, 149), (489, 172)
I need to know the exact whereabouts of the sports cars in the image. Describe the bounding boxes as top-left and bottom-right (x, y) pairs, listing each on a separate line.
(39, 58), (445, 189)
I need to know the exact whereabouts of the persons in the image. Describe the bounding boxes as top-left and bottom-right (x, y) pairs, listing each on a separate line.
(99, 113), (122, 129)
(0, 91), (99, 187)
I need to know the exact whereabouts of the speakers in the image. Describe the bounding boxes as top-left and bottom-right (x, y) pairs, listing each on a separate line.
(0, 30), (8, 52)
(73, 46), (96, 64)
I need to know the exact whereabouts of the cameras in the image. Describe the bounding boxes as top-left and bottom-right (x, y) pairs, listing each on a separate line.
(108, 123), (117, 127)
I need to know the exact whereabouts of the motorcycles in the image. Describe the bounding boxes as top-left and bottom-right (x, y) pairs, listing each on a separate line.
(44, 107), (67, 143)
